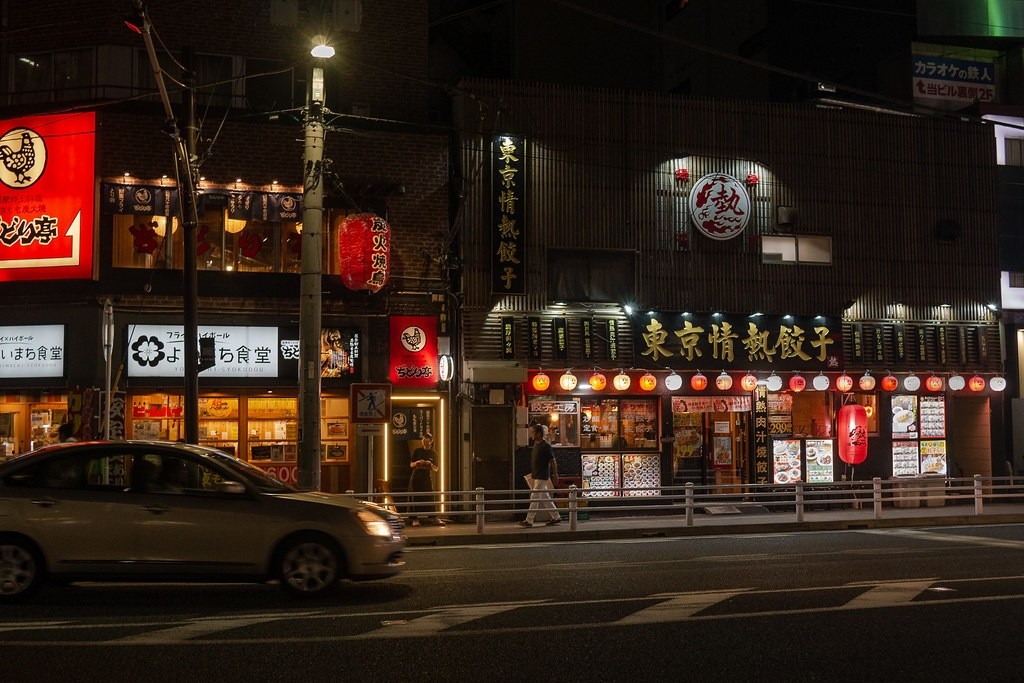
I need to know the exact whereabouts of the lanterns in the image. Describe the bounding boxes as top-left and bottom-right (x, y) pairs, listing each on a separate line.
(692, 372), (853, 391)
(533, 372), (683, 391)
(838, 400), (868, 466)
(337, 212), (391, 301)
(859, 373), (1007, 392)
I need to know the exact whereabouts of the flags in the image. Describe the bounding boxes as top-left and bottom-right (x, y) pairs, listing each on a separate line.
(388, 315), (439, 388)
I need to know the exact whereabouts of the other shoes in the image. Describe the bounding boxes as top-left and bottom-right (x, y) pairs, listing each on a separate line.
(546, 519), (561, 526)
(412, 520), (421, 527)
(434, 519), (445, 526)
(518, 520), (532, 527)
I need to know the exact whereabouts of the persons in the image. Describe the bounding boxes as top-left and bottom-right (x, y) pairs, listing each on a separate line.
(57, 423), (77, 443)
(519, 424), (563, 527)
(406, 433), (447, 527)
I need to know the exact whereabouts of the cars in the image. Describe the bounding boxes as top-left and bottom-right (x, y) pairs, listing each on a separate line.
(0, 439), (409, 602)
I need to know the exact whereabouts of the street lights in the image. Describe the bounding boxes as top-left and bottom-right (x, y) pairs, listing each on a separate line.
(295, 32), (337, 492)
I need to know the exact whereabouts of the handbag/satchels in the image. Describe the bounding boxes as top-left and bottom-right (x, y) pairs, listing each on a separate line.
(524, 473), (554, 489)
